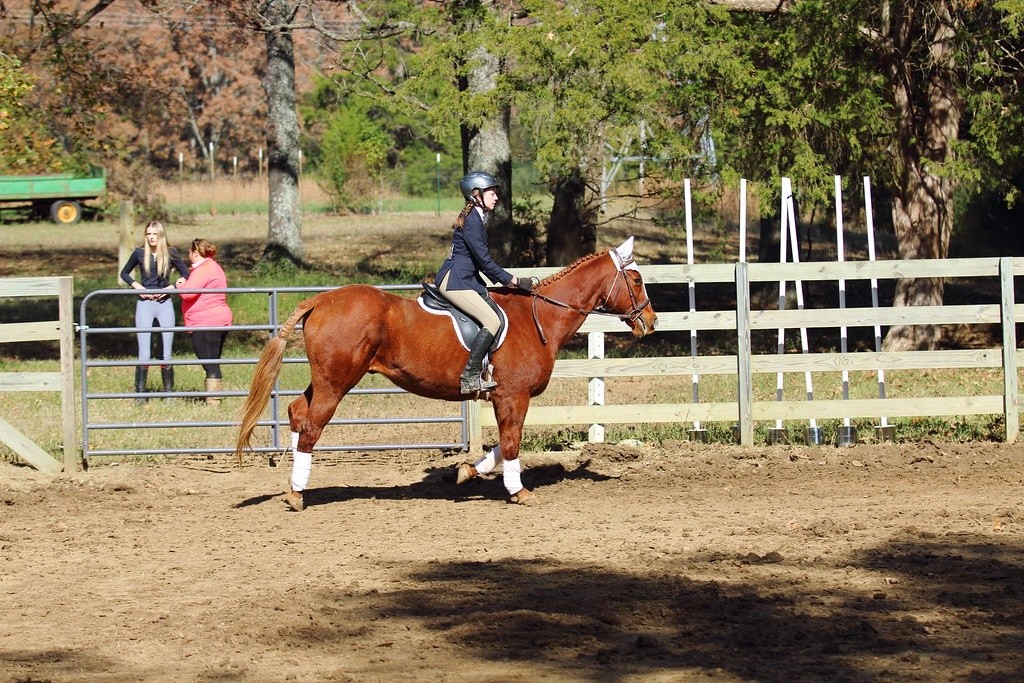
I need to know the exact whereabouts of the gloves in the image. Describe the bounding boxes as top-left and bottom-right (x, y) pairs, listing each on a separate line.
(514, 277), (533, 292)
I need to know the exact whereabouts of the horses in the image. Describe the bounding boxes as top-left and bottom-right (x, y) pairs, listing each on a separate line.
(227, 235), (657, 512)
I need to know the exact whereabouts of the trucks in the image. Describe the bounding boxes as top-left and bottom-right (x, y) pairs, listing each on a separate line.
(0, 160), (108, 225)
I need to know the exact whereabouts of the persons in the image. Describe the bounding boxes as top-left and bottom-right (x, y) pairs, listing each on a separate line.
(435, 171), (533, 394)
(176, 239), (232, 405)
(120, 220), (191, 405)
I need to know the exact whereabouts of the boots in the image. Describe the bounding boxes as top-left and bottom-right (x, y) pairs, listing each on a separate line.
(161, 365), (174, 403)
(135, 365), (148, 404)
(205, 378), (223, 406)
(460, 328), (498, 394)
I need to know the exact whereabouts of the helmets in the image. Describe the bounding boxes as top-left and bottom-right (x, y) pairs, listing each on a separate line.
(459, 172), (500, 201)
